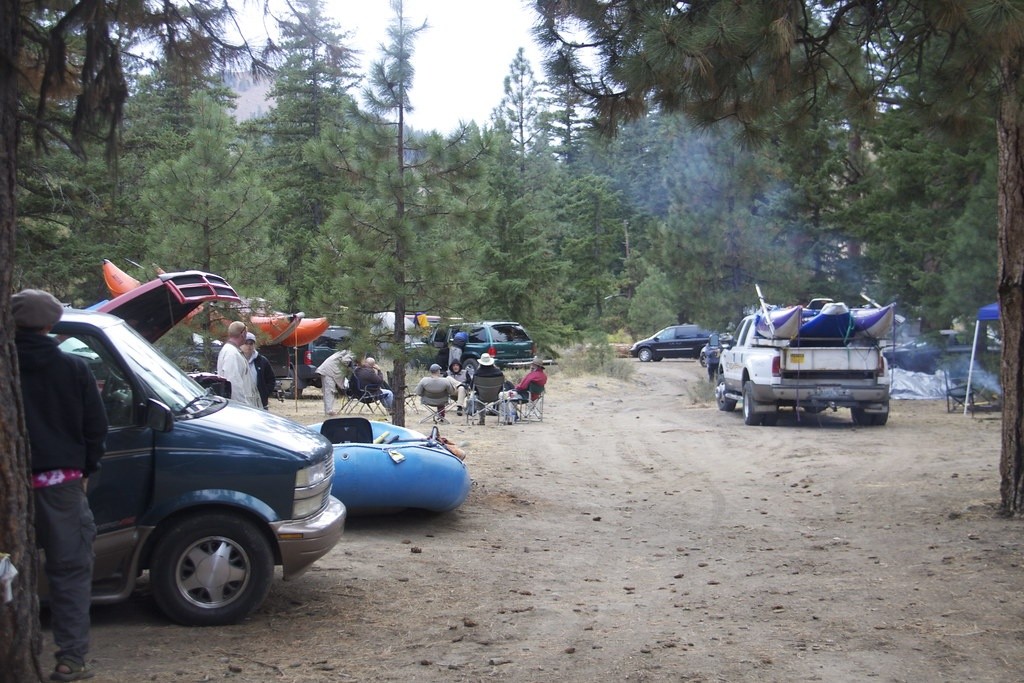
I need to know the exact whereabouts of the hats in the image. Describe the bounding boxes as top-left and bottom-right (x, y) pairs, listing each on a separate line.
(13, 289), (63, 330)
(532, 359), (545, 371)
(430, 364), (443, 372)
(477, 353), (495, 366)
(245, 331), (257, 343)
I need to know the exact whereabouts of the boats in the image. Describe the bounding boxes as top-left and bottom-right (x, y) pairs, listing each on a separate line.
(99, 258), (335, 348)
(753, 302), (899, 340)
(303, 416), (470, 522)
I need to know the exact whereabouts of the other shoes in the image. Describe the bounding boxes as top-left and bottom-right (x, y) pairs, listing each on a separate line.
(503, 421), (513, 425)
(457, 409), (463, 416)
(327, 411), (337, 416)
(477, 422), (484, 426)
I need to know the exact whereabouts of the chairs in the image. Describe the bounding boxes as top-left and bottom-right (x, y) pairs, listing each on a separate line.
(335, 371), (545, 427)
(320, 417), (373, 444)
(943, 368), (976, 413)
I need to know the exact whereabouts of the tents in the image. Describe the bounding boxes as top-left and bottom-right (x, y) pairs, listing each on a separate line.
(962, 303), (1003, 417)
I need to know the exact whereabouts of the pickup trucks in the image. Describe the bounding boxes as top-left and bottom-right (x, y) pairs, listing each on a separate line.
(156, 338), (291, 385)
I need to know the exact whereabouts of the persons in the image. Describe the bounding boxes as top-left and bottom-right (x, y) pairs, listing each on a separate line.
(446, 328), (468, 372)
(10, 288), (109, 683)
(414, 363), (454, 422)
(504, 357), (548, 417)
(705, 334), (725, 384)
(353, 357), (393, 415)
(240, 331), (276, 410)
(314, 348), (356, 415)
(443, 358), (471, 416)
(468, 352), (505, 415)
(217, 321), (264, 410)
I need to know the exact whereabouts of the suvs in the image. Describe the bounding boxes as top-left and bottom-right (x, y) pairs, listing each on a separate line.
(700, 298), (891, 426)
(404, 322), (537, 370)
(46, 308), (348, 629)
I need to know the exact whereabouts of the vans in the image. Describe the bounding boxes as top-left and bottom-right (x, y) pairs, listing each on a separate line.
(272, 326), (353, 399)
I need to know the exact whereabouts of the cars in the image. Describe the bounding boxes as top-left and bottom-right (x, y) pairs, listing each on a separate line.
(628, 325), (711, 362)
(79, 258), (242, 401)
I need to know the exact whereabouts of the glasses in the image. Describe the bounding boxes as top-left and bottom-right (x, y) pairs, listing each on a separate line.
(245, 341), (253, 345)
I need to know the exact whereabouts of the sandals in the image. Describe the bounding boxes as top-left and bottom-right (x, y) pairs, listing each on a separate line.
(50, 654), (93, 682)
